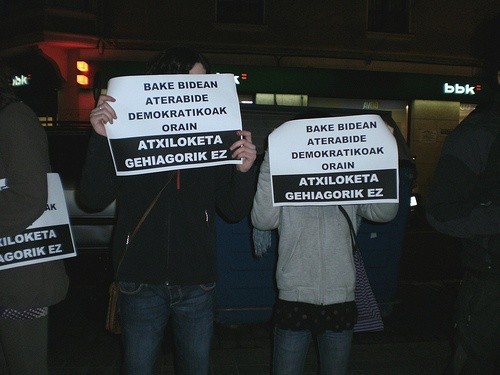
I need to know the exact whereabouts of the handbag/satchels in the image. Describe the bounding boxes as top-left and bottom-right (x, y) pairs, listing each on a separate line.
(354, 247), (385, 333)
(104, 282), (122, 334)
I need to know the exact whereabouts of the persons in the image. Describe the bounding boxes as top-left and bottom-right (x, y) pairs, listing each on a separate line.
(80, 50), (256, 375)
(426, 69), (500, 375)
(251, 122), (398, 375)
(0, 92), (71, 375)
